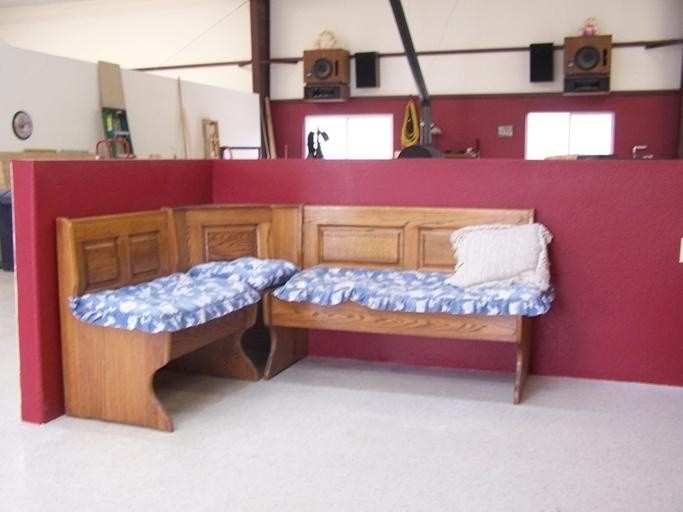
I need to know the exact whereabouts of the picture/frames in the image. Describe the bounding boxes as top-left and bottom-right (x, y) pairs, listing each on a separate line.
(202, 118), (221, 160)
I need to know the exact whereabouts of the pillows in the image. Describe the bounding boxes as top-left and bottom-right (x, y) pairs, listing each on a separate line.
(444, 223), (553, 290)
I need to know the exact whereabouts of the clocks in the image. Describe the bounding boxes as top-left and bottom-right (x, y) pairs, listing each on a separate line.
(13, 111), (32, 140)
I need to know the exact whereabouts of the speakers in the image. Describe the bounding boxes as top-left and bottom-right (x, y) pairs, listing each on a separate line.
(355, 51), (378, 88)
(304, 49), (351, 104)
(530, 43), (554, 82)
(563, 34), (612, 96)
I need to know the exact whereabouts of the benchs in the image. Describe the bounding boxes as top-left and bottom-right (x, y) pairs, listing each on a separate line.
(58, 206), (264, 432)
(260, 204), (552, 404)
